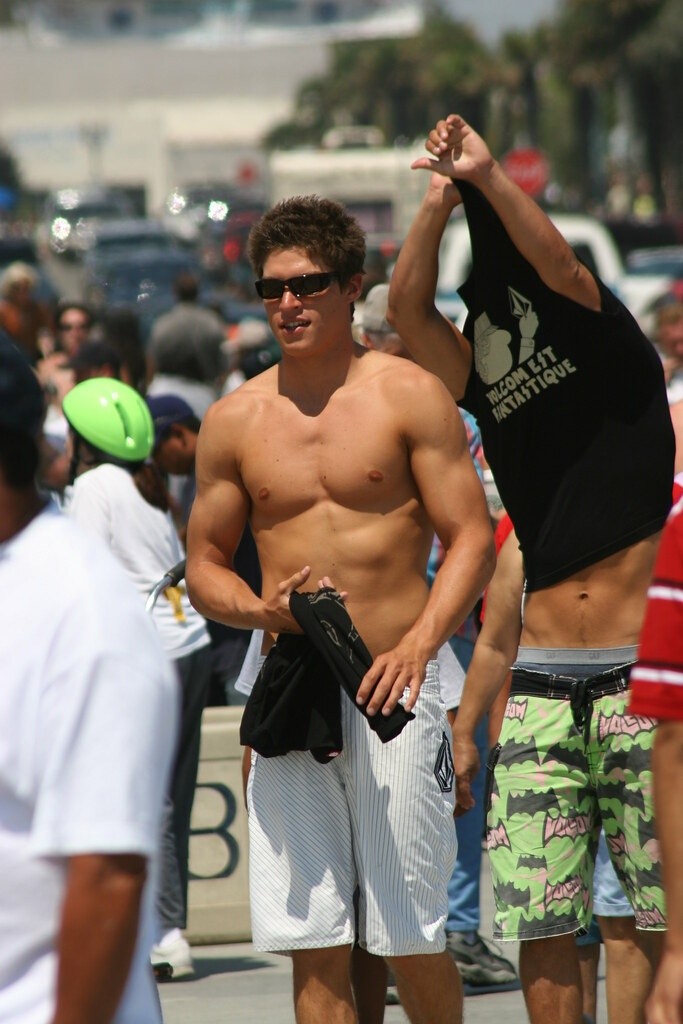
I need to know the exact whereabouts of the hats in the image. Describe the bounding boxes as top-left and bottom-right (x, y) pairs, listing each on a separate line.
(146, 396), (193, 447)
(56, 344), (120, 372)
(360, 285), (396, 334)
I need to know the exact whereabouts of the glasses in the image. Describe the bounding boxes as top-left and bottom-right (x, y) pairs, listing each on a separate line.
(255, 271), (340, 299)
(59, 322), (93, 331)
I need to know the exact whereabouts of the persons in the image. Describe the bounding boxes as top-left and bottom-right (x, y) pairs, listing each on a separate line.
(629, 495), (683, 1024)
(0, 264), (683, 1000)
(0, 330), (175, 1024)
(387, 114), (677, 1024)
(185, 195), (498, 1024)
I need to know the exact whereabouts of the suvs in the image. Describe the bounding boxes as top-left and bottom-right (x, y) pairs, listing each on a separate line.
(267, 125), (440, 290)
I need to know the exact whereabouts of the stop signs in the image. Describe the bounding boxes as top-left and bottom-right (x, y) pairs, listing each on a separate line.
(503, 152), (548, 195)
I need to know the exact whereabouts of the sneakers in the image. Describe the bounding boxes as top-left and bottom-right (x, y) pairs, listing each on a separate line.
(384, 972), (401, 1005)
(445, 932), (520, 995)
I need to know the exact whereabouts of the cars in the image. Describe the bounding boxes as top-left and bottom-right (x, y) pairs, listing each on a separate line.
(357, 214), (683, 402)
(43, 183), (284, 371)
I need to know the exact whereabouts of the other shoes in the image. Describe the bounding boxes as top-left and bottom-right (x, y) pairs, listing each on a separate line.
(148, 938), (195, 979)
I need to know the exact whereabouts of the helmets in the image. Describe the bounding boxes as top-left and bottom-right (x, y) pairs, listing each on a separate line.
(63, 377), (154, 461)
